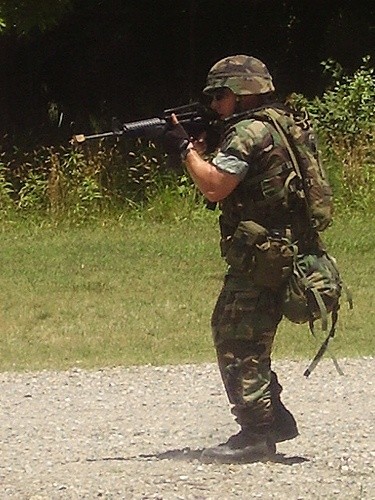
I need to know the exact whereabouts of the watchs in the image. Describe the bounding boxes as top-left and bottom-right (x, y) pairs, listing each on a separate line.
(180, 147), (195, 160)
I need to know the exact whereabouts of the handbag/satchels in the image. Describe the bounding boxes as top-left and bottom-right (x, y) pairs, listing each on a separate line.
(283, 245), (343, 324)
(227, 221), (298, 287)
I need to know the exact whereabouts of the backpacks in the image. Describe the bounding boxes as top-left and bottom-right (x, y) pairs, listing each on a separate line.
(222, 106), (332, 230)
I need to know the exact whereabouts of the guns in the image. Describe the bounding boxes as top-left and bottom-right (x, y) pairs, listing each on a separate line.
(72, 102), (219, 169)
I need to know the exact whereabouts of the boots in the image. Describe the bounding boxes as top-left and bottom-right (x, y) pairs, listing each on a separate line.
(199, 418), (276, 464)
(271, 382), (298, 444)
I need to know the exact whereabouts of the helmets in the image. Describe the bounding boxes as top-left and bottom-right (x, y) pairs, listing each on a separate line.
(202, 55), (274, 94)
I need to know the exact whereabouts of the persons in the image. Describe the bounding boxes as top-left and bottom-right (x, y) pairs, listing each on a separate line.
(165, 55), (301, 463)
